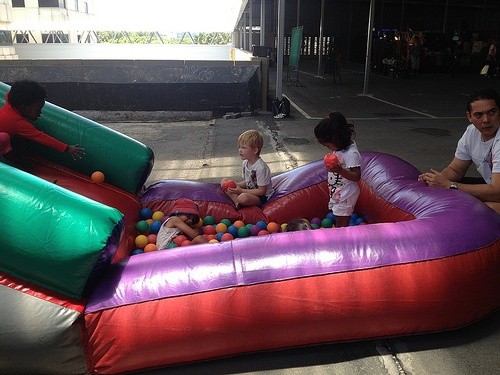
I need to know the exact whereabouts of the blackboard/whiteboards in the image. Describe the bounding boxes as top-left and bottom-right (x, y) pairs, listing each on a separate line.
(287, 26), (304, 68)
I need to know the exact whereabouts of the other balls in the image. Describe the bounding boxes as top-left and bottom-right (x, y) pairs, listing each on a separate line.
(131, 208), (368, 255)
(223, 180), (237, 191)
(91, 171), (104, 183)
(324, 153), (339, 165)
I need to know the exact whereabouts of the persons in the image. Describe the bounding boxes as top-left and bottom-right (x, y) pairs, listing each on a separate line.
(410, 34), (420, 73)
(221, 130), (275, 210)
(417, 92), (500, 215)
(400, 35), (408, 68)
(487, 39), (497, 80)
(156, 198), (208, 251)
(0, 79), (85, 171)
(314, 111), (361, 227)
(284, 218), (315, 232)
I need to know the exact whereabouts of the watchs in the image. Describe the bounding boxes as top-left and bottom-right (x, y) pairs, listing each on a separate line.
(449, 182), (458, 190)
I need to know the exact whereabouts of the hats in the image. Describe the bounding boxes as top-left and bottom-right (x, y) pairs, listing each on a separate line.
(169, 198), (200, 224)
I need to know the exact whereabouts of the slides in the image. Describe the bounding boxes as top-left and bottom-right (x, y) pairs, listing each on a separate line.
(0, 80), (155, 350)
